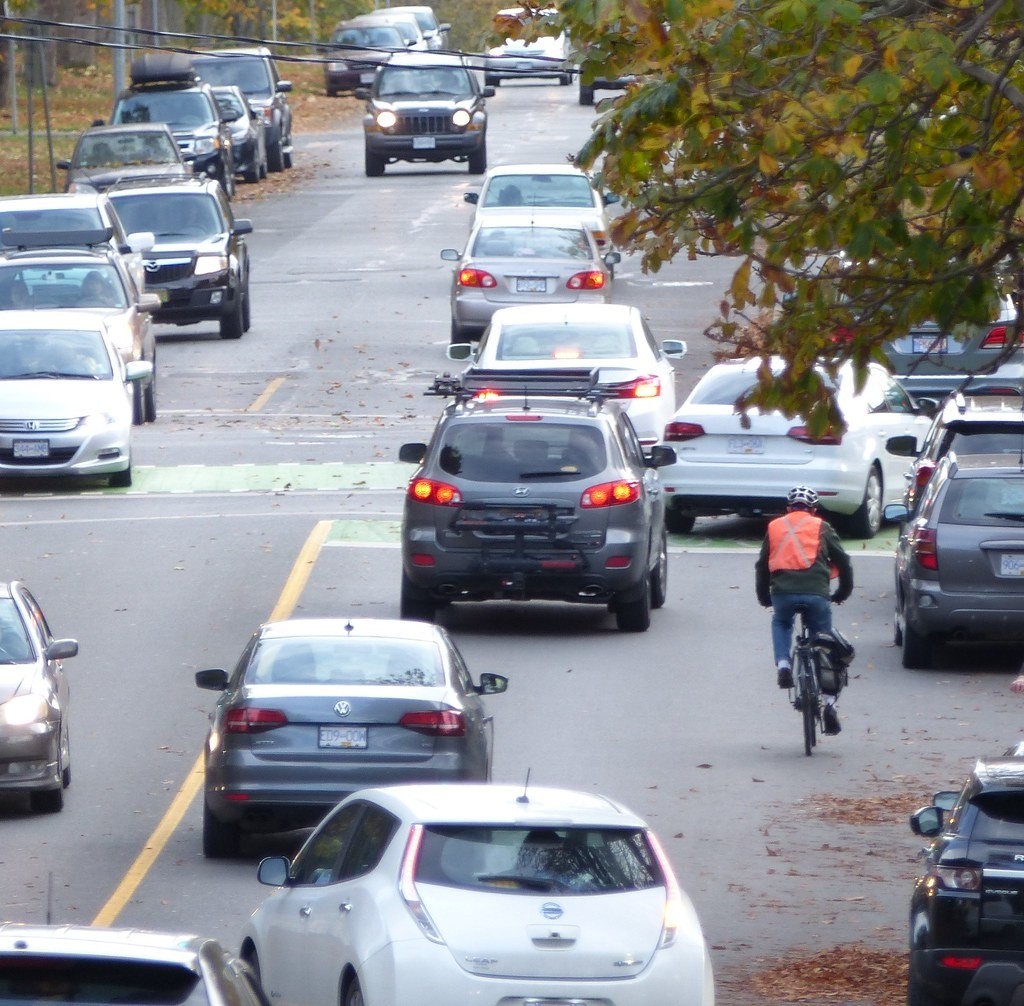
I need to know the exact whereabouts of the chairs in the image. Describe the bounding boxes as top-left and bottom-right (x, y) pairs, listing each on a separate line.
(272, 646), (318, 682)
(91, 142), (116, 161)
(488, 239), (511, 257)
(512, 336), (538, 356)
(516, 831), (567, 876)
(595, 335), (622, 353)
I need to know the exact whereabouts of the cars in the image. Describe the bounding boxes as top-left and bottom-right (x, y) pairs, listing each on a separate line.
(446, 303), (689, 467)
(782, 247), (1024, 396)
(657, 355), (935, 540)
(907, 738), (1024, 1006)
(0, 923), (267, 1005)
(0, 579), (79, 813)
(441, 163), (620, 344)
(485, 8), (671, 104)
(238, 783), (714, 1005)
(316, 6), (451, 97)
(195, 619), (509, 860)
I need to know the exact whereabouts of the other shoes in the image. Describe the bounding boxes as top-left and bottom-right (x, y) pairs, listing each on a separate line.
(778, 668), (793, 688)
(824, 707), (841, 735)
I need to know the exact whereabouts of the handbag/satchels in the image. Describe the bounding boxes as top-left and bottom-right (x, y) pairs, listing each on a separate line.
(814, 627), (855, 694)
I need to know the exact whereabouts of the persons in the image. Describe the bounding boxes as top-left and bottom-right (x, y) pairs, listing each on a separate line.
(178, 201), (207, 232)
(755, 483), (854, 736)
(72, 345), (96, 369)
(81, 270), (115, 307)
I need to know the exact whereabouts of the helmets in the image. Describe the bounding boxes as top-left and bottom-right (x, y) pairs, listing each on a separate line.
(787, 486), (819, 508)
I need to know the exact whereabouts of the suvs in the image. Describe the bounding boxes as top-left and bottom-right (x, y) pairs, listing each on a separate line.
(355, 52), (495, 176)
(398, 368), (679, 633)
(0, 45), (293, 488)
(883, 393), (1024, 671)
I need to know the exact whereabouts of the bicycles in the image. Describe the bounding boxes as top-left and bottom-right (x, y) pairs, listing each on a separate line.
(772, 592), (844, 754)
